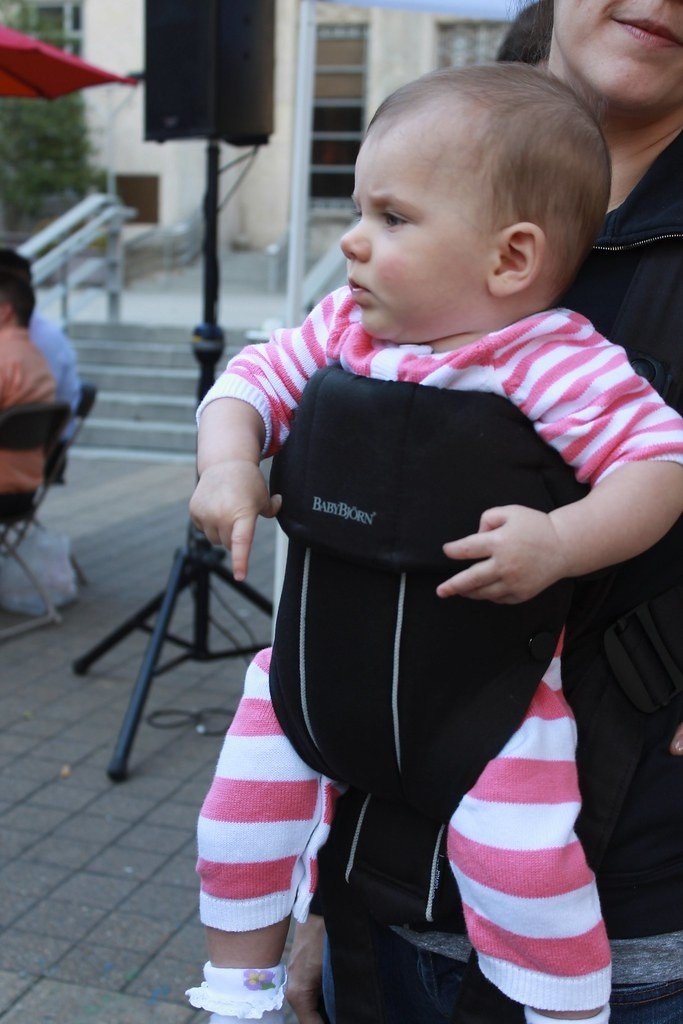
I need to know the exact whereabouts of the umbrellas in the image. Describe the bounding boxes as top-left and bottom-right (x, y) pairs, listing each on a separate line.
(1, 24), (138, 103)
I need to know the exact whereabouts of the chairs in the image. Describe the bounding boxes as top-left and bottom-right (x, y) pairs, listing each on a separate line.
(0, 381), (97, 645)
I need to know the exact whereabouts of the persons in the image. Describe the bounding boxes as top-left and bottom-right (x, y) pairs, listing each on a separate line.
(184, 66), (682, 1024)
(291, 0), (683, 1024)
(1, 250), (81, 525)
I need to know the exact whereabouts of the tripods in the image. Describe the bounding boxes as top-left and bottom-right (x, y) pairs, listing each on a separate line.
(72, 141), (274, 781)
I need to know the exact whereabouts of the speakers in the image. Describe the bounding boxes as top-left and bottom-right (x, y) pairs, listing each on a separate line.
(144, 0), (275, 146)
